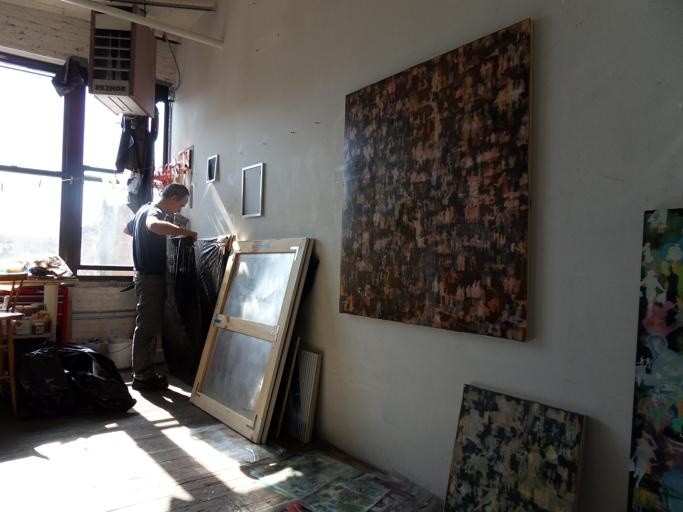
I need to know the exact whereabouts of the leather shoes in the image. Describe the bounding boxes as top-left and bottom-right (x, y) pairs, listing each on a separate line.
(133, 374), (169, 393)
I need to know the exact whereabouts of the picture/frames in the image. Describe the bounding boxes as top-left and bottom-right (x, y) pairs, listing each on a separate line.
(281, 341), (321, 444)
(444, 385), (586, 512)
(337, 17), (536, 343)
(625, 206), (683, 512)
(175, 145), (194, 208)
(206, 155), (217, 184)
(241, 162), (262, 218)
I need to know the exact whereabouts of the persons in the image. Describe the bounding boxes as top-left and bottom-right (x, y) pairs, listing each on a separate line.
(122, 183), (198, 391)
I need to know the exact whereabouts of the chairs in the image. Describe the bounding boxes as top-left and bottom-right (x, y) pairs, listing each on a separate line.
(0, 273), (27, 426)
